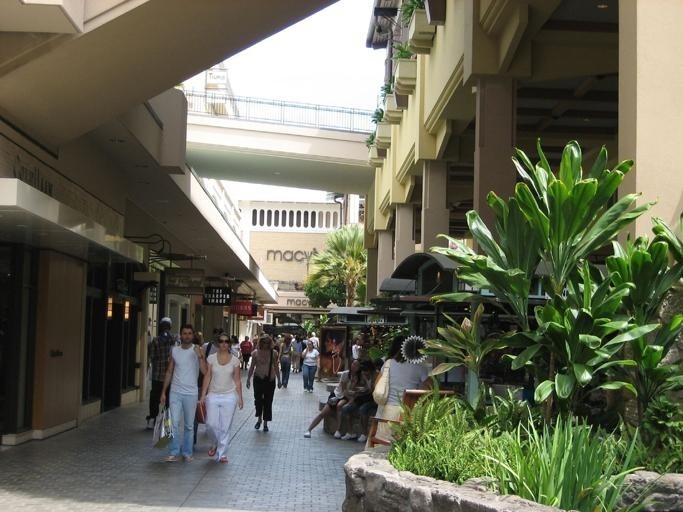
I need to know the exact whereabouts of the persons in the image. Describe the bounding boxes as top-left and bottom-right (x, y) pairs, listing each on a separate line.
(373, 336), (432, 445)
(351, 339), (362, 359)
(192, 331), (204, 344)
(246, 333), (281, 432)
(303, 360), (361, 438)
(229, 330), (321, 393)
(329, 338), (342, 374)
(199, 327), (234, 356)
(200, 331), (243, 464)
(341, 359), (384, 443)
(147, 316), (177, 429)
(159, 324), (207, 463)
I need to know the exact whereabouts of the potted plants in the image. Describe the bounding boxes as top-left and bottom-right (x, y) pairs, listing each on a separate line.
(366, 1), (437, 169)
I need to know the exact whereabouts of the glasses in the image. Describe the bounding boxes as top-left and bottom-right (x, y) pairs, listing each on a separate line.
(218, 339), (230, 344)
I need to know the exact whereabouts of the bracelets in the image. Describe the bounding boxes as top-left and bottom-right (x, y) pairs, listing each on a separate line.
(199, 354), (203, 359)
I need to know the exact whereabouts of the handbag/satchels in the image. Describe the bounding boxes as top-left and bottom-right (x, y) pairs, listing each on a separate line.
(198, 368), (205, 388)
(371, 358), (392, 406)
(152, 403), (175, 450)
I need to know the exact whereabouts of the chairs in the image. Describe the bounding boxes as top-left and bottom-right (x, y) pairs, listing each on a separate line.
(363, 387), (455, 448)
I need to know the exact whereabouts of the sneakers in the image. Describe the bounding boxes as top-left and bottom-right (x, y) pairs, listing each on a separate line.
(263, 426), (268, 431)
(334, 430), (341, 439)
(255, 420), (262, 429)
(304, 388), (313, 393)
(183, 455), (193, 464)
(208, 445), (217, 456)
(219, 456), (228, 463)
(357, 433), (367, 442)
(160, 454), (182, 462)
(341, 432), (357, 440)
(304, 430), (311, 437)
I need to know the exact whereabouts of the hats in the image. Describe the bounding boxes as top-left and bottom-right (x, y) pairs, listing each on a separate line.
(160, 317), (173, 328)
